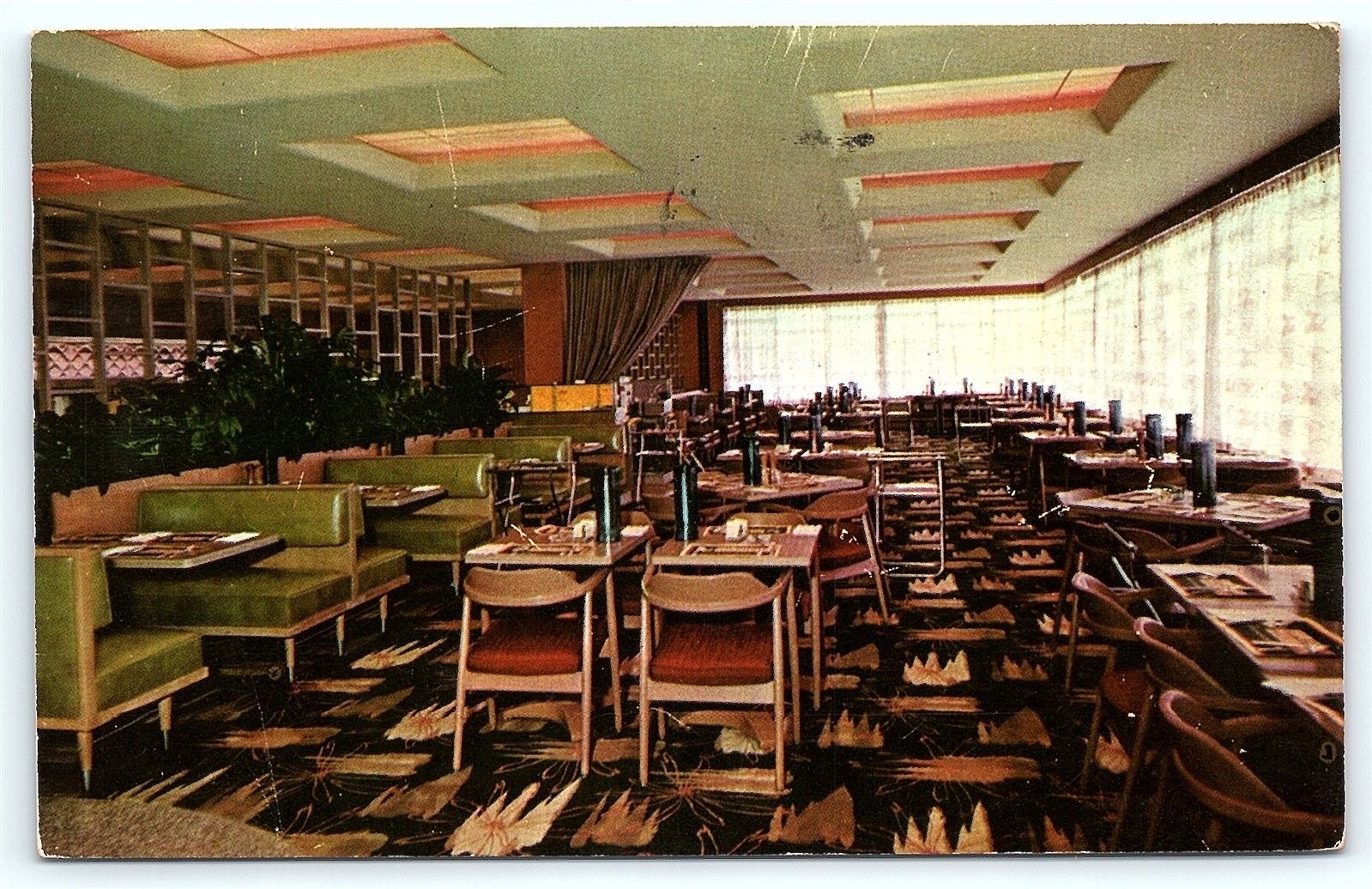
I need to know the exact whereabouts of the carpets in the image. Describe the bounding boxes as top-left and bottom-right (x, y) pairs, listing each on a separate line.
(37, 796), (306, 857)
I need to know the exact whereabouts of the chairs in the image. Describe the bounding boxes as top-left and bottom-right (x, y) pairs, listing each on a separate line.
(442, 405), (1345, 854)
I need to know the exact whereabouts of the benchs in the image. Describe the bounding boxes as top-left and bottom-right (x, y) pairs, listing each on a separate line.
(35, 411), (632, 789)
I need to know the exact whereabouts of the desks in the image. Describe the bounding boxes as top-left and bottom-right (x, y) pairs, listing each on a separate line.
(50, 393), (1343, 744)
